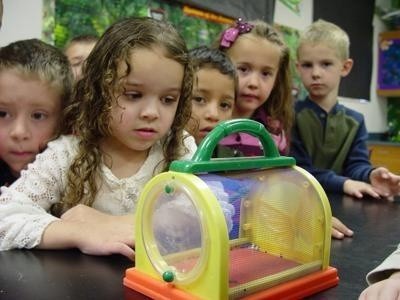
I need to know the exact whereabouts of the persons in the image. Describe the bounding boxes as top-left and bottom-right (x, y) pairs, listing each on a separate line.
(1, 15), (400, 299)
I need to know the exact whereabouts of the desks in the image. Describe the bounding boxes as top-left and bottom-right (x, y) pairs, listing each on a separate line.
(0, 194), (400, 300)
